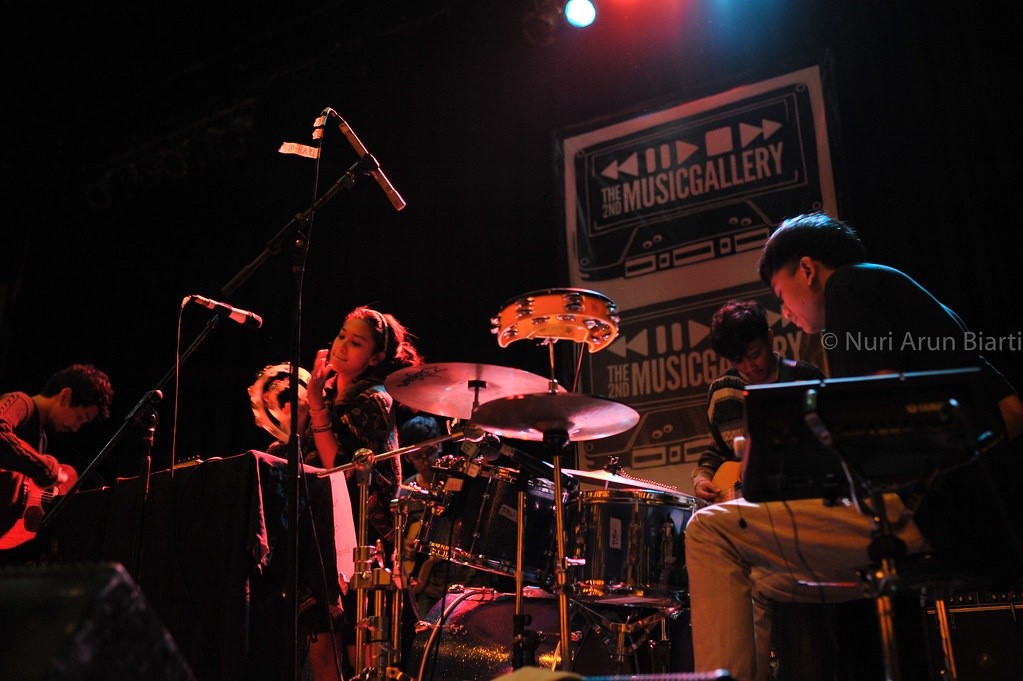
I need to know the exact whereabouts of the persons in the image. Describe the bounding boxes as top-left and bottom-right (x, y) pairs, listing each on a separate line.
(689, 300), (827, 504)
(306, 305), (426, 681)
(265, 402), (292, 457)
(0, 364), (113, 566)
(684, 211), (1023, 681)
(400, 416), (504, 620)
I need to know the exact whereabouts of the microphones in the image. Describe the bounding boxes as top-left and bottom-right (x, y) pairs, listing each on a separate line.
(332, 111), (406, 211)
(489, 441), (579, 493)
(193, 294), (263, 329)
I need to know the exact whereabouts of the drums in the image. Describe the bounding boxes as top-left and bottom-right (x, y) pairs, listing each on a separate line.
(566, 488), (698, 605)
(406, 583), (617, 681)
(413, 451), (569, 592)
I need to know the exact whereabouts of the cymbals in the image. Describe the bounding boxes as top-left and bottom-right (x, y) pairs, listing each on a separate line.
(471, 392), (640, 441)
(561, 469), (709, 504)
(384, 362), (569, 419)
(490, 287), (621, 354)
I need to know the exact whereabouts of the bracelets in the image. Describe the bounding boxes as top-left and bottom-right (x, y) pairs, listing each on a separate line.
(311, 421), (333, 432)
(309, 405), (329, 417)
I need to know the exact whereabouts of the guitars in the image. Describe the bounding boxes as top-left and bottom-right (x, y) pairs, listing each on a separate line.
(0, 454), (226, 550)
